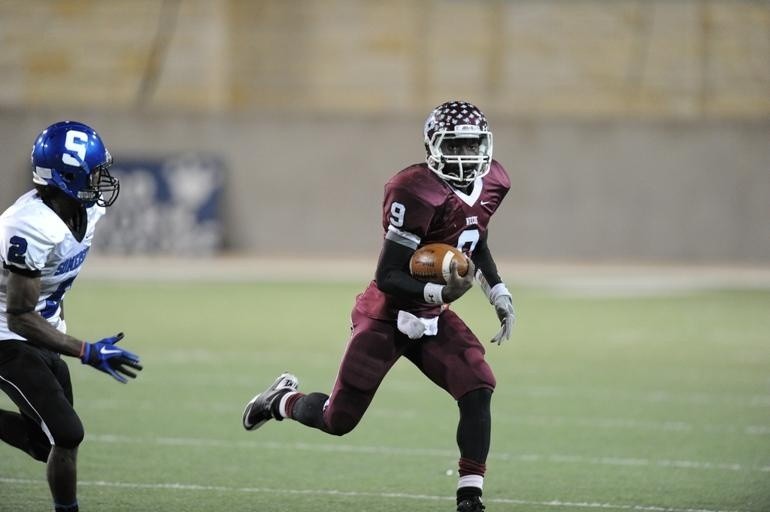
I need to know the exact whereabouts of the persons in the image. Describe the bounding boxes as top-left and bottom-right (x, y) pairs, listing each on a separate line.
(0, 121), (143, 510)
(243, 101), (514, 512)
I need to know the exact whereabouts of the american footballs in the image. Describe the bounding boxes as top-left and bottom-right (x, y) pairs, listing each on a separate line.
(410, 243), (467, 283)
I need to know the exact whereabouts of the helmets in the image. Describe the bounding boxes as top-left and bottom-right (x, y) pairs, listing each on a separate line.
(29, 119), (120, 211)
(421, 100), (494, 184)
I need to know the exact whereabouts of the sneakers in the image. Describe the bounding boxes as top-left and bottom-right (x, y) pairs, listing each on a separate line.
(239, 371), (299, 433)
(455, 485), (487, 511)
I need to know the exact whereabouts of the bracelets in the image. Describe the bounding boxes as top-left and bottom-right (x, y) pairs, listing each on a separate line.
(423, 282), (444, 306)
(79, 340), (85, 359)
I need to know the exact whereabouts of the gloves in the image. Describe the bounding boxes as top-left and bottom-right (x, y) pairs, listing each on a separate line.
(84, 331), (145, 385)
(484, 283), (530, 352)
